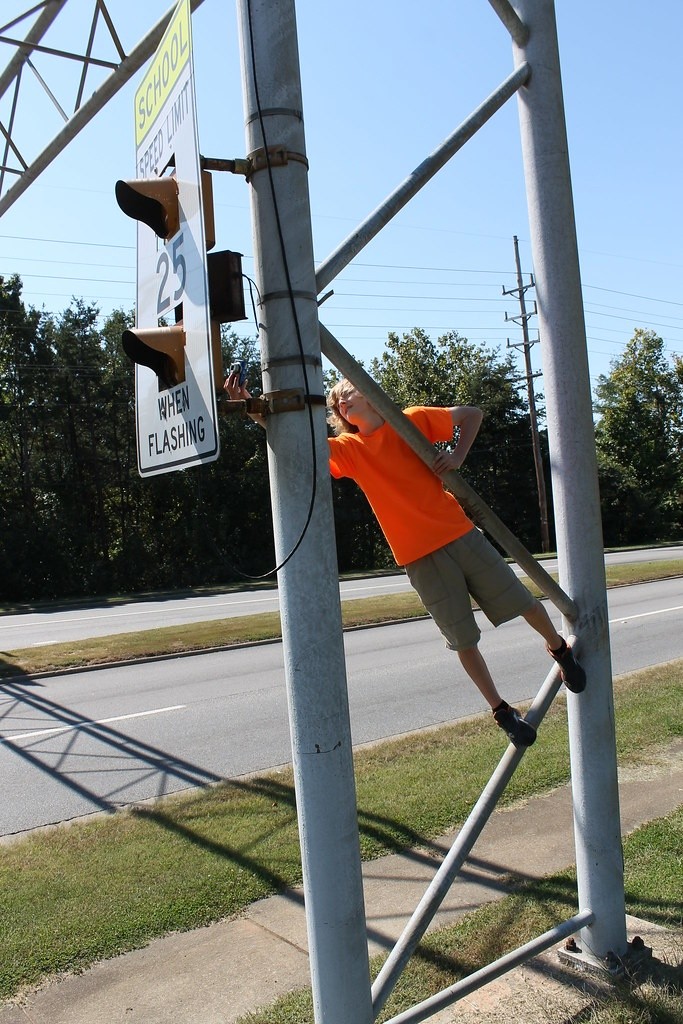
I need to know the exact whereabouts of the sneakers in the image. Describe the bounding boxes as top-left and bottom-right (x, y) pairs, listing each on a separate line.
(493, 705), (537, 746)
(544, 634), (587, 693)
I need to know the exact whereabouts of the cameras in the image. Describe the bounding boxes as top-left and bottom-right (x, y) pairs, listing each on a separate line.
(230, 359), (248, 387)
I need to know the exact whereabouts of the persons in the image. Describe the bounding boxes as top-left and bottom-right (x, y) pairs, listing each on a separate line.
(223, 371), (588, 749)
